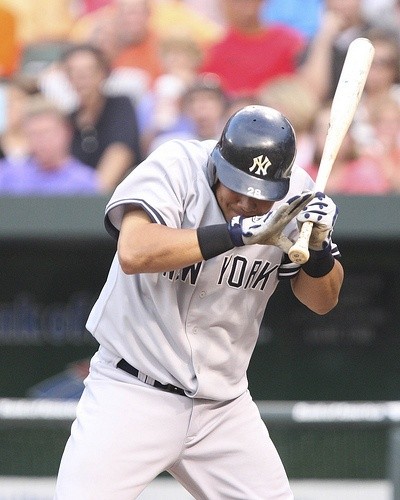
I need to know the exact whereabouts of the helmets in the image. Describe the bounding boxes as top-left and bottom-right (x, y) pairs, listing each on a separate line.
(211, 105), (296, 200)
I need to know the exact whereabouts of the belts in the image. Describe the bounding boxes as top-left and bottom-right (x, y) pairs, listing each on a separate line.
(116, 358), (187, 397)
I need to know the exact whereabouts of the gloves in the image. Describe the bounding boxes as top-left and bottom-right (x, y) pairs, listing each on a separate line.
(296, 189), (339, 251)
(229, 194), (313, 256)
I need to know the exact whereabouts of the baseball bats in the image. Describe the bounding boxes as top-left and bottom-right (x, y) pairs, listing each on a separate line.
(288, 37), (375, 264)
